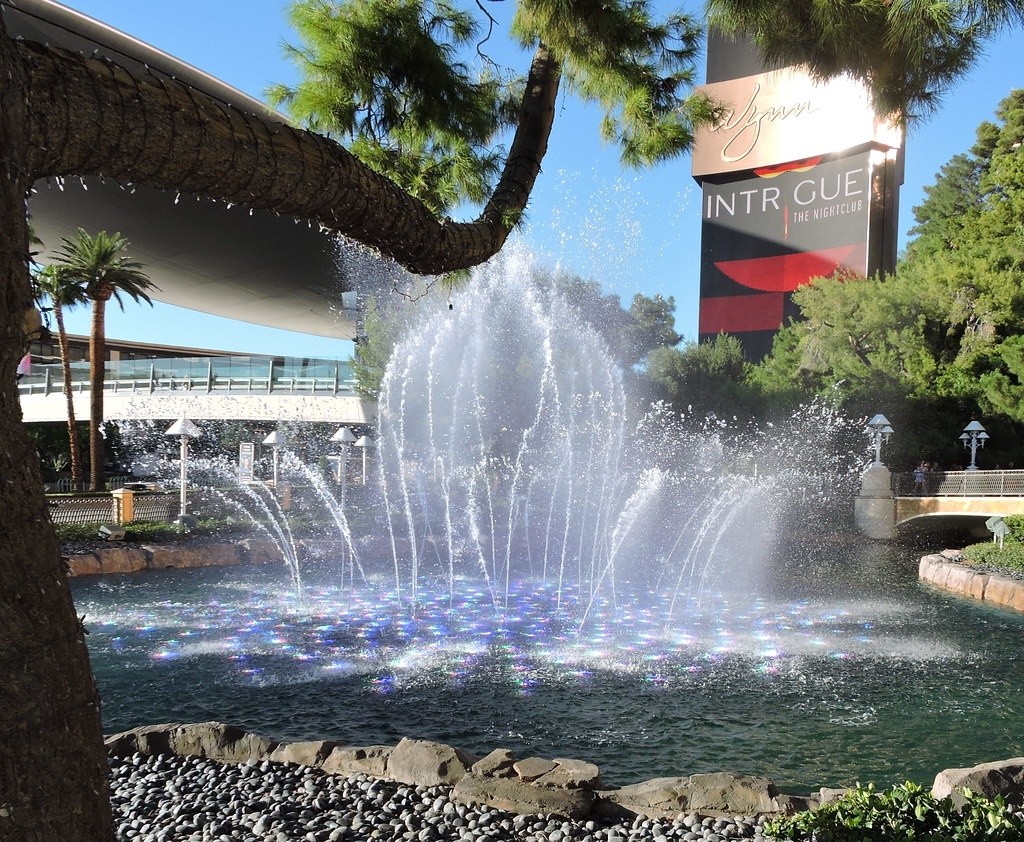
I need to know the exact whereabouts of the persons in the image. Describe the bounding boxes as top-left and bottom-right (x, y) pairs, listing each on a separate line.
(913, 460), (929, 495)
(930, 462), (939, 473)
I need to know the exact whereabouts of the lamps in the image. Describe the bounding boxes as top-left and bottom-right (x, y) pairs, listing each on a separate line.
(98, 524), (126, 540)
(986, 517), (1012, 537)
